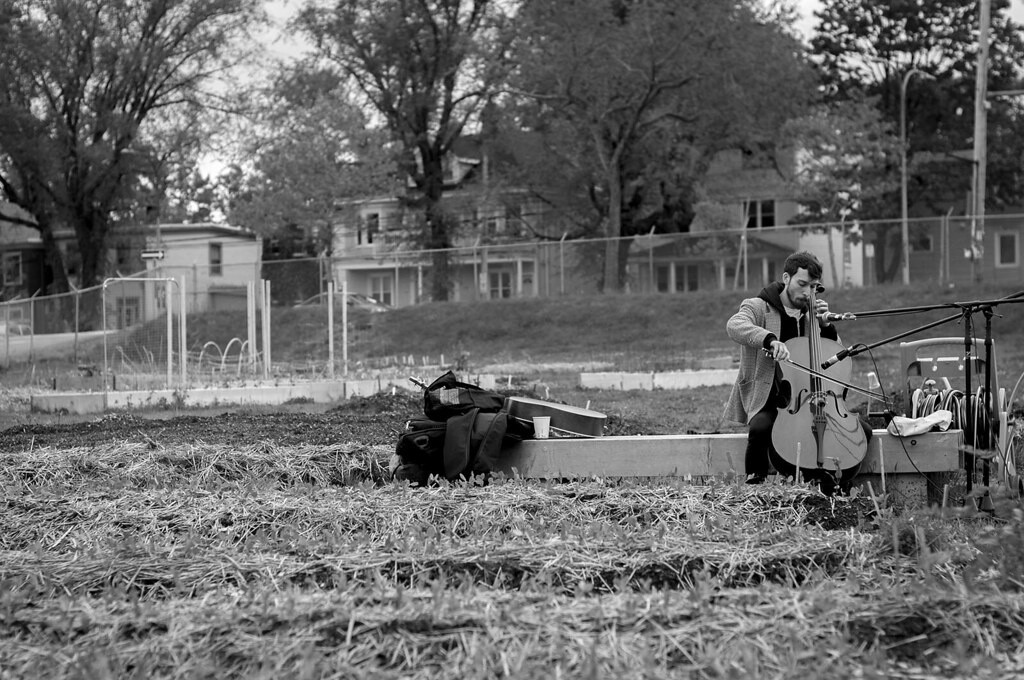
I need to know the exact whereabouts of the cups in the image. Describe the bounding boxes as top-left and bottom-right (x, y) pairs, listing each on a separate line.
(532, 416), (551, 439)
(868, 372), (880, 389)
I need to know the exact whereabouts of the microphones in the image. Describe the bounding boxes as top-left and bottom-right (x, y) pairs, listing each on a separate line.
(821, 311), (847, 322)
(821, 343), (860, 370)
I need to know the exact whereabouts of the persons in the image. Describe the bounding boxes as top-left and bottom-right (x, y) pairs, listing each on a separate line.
(726, 252), (873, 484)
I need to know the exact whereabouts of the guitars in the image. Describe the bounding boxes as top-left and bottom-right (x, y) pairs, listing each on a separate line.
(504, 396), (607, 437)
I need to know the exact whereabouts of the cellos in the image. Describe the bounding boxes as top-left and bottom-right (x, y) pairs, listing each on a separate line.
(768, 278), (867, 488)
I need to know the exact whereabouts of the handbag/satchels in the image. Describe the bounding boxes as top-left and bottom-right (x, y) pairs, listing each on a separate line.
(888, 410), (952, 436)
(424, 370), (502, 420)
